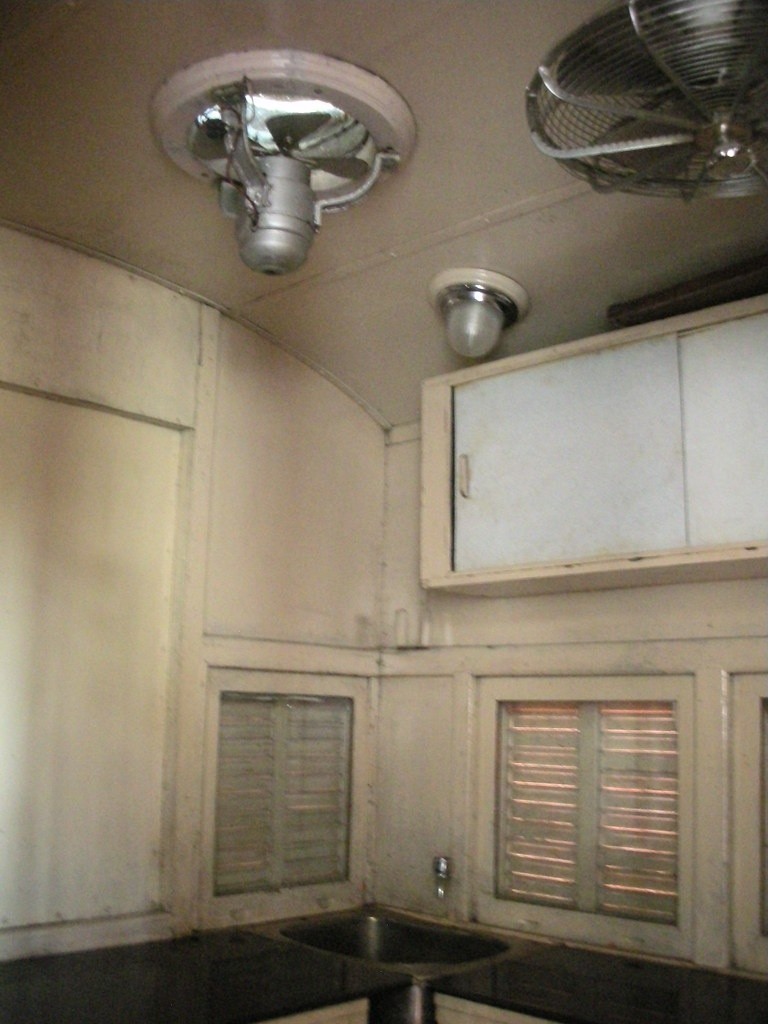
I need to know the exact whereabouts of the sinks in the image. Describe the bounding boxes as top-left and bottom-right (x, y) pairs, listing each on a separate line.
(278, 911), (511, 968)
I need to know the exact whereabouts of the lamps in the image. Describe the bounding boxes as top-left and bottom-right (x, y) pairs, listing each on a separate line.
(428, 269), (528, 359)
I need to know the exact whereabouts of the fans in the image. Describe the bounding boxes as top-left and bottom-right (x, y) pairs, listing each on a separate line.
(522, 0), (768, 205)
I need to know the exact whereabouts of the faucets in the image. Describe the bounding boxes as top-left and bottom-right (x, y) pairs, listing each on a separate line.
(432, 853), (454, 903)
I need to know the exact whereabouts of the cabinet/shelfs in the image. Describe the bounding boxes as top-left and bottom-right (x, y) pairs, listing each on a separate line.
(418, 292), (768, 598)
